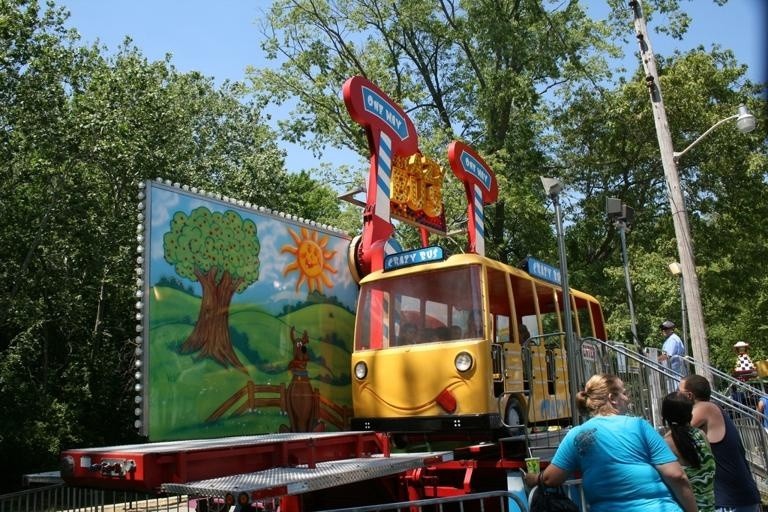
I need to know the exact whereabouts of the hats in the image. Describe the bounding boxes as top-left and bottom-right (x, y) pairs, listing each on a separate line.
(659, 320), (675, 329)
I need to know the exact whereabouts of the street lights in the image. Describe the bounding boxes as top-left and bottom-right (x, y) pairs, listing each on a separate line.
(540, 176), (579, 427)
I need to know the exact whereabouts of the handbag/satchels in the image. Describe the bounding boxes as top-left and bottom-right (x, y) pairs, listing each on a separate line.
(527, 470), (584, 512)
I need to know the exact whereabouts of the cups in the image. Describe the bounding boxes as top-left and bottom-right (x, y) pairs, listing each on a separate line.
(525, 457), (540, 474)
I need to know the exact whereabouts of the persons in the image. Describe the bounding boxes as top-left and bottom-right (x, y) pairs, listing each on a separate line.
(465, 307), (483, 338)
(658, 389), (717, 511)
(756, 394), (768, 485)
(430, 326), (450, 339)
(664, 375), (765, 511)
(398, 321), (418, 343)
(658, 319), (687, 397)
(518, 322), (529, 344)
(521, 374), (703, 511)
(448, 325), (462, 339)
